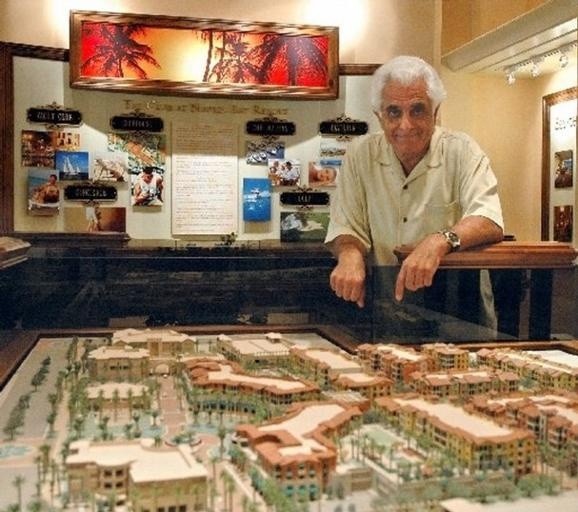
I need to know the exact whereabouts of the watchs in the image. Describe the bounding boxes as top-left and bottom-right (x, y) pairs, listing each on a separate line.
(437, 225), (463, 253)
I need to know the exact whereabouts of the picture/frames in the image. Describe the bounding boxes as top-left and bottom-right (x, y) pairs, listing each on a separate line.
(540, 87), (578, 251)
(68, 8), (340, 101)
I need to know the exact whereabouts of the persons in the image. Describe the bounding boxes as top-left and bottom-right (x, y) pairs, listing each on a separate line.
(130, 166), (164, 206)
(268, 160), (300, 187)
(321, 55), (507, 340)
(30, 174), (60, 204)
(309, 162), (338, 187)
